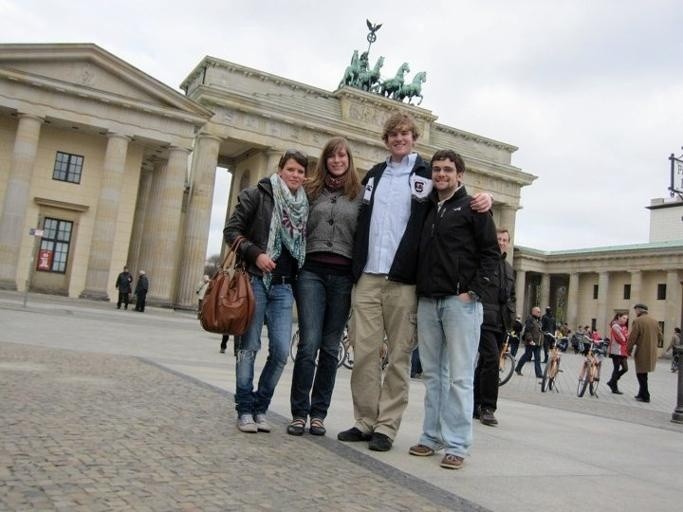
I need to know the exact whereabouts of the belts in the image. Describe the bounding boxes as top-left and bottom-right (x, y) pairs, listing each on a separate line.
(270, 276), (297, 285)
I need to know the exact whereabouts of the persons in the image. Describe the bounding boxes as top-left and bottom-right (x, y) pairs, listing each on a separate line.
(471, 228), (516, 426)
(195, 274), (210, 319)
(286, 136), (365, 435)
(409, 150), (500, 470)
(131, 270), (149, 312)
(624, 303), (662, 402)
(334, 112), (494, 451)
(666, 327), (681, 373)
(222, 148), (310, 433)
(115, 266), (133, 310)
(507, 307), (603, 378)
(219, 335), (239, 356)
(607, 310), (631, 394)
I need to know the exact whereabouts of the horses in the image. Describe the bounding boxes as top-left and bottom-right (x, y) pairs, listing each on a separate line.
(358, 55), (385, 92)
(381, 62), (410, 98)
(395, 72), (427, 105)
(338, 49), (361, 88)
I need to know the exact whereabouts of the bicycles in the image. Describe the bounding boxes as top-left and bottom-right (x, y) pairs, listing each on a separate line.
(498, 330), (519, 387)
(539, 328), (570, 396)
(577, 332), (609, 400)
(291, 325), (389, 369)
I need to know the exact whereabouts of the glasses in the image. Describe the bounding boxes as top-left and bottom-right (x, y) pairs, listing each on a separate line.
(537, 311), (540, 313)
(282, 148), (308, 159)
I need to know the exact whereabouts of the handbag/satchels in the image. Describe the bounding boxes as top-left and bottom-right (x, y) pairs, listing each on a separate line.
(128, 287), (131, 293)
(506, 302), (516, 329)
(197, 266), (255, 337)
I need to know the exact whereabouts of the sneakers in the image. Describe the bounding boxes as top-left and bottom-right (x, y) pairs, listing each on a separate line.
(338, 427), (374, 441)
(410, 438), (441, 458)
(370, 428), (394, 451)
(117, 305), (120, 308)
(124, 306), (127, 310)
(441, 445), (469, 470)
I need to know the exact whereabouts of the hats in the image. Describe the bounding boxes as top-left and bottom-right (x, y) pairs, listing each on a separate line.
(633, 304), (647, 310)
(124, 266), (128, 269)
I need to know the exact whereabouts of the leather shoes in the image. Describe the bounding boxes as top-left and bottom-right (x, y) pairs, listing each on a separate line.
(253, 411), (272, 432)
(514, 368), (523, 376)
(138, 310), (144, 312)
(472, 403), (481, 420)
(287, 413), (307, 435)
(236, 412), (258, 433)
(480, 406), (498, 425)
(310, 413), (326, 435)
(611, 390), (623, 395)
(132, 308), (139, 311)
(606, 381), (613, 390)
(637, 397), (650, 403)
(634, 395), (641, 398)
(536, 375), (544, 378)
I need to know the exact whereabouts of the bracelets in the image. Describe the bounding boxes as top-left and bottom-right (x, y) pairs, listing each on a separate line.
(468, 290), (480, 300)
